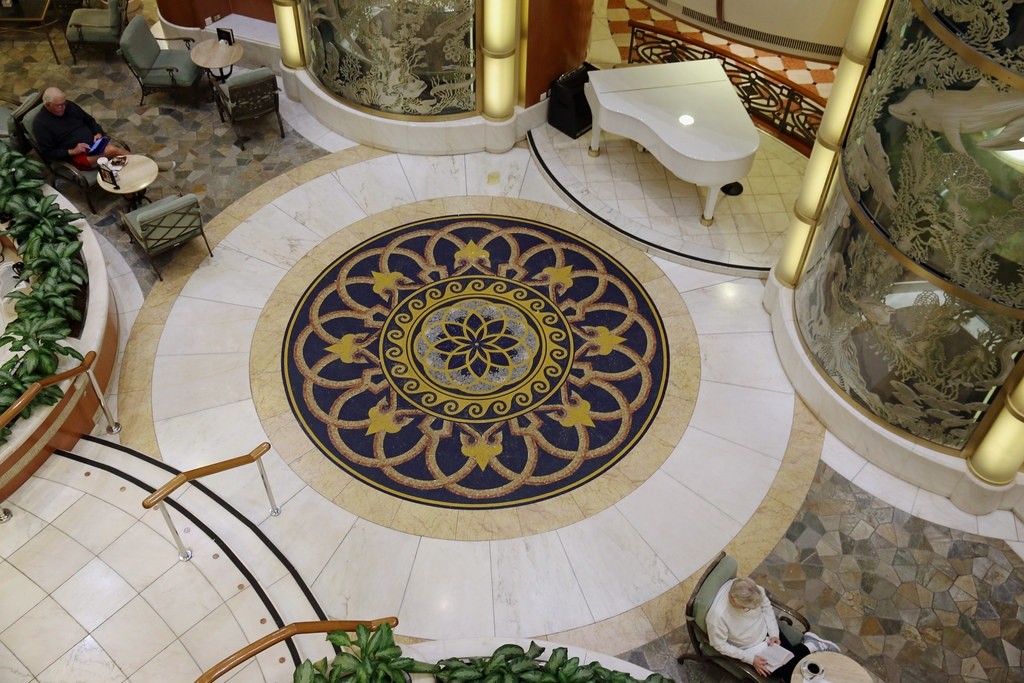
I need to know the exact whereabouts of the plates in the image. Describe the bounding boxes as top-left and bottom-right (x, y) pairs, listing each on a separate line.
(109, 156), (128, 170)
(801, 659), (825, 681)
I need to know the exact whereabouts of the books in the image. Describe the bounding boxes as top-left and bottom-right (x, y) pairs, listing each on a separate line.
(744, 636), (795, 673)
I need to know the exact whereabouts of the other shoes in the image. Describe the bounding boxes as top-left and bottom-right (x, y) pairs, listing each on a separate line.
(156, 161), (176, 171)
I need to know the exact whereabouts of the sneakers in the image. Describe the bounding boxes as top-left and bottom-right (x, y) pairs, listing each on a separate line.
(803, 632), (841, 653)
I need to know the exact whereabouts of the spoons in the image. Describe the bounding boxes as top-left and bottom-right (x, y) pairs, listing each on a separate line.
(810, 670), (824, 680)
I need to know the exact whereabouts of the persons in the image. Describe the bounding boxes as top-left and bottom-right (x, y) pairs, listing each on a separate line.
(705, 577), (841, 683)
(32, 87), (176, 199)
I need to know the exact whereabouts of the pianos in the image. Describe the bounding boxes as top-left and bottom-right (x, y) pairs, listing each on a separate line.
(582, 57), (760, 226)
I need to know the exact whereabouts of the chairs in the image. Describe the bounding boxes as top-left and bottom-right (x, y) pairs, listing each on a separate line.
(20, 103), (131, 214)
(677, 551), (811, 683)
(117, 185), (213, 281)
(0, 91), (45, 157)
(117, 14), (204, 108)
(209, 65), (285, 151)
(66, 0), (129, 63)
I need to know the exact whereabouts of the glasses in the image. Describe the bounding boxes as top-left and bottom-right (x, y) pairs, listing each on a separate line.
(743, 608), (750, 612)
(47, 102), (66, 108)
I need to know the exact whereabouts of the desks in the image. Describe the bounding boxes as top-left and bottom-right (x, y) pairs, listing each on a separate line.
(790, 651), (874, 683)
(97, 154), (158, 213)
(0, 0), (66, 65)
(190, 38), (244, 83)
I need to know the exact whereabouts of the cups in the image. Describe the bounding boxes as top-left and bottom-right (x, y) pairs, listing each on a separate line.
(97, 157), (109, 169)
(803, 660), (822, 676)
